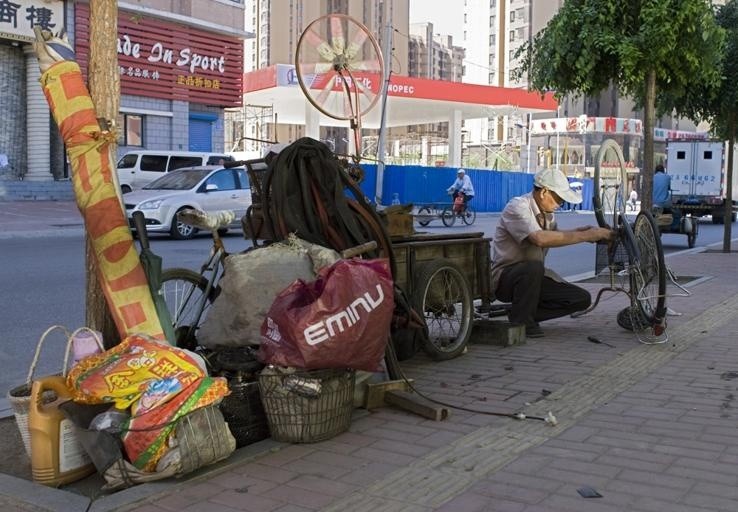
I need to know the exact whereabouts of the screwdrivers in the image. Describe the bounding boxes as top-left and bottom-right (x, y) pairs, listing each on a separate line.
(587, 336), (617, 348)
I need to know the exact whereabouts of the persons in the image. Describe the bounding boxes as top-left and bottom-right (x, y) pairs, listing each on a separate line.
(446, 168), (475, 218)
(560, 200), (571, 211)
(489, 165), (623, 339)
(628, 188), (638, 212)
(652, 164), (681, 232)
(572, 186), (582, 211)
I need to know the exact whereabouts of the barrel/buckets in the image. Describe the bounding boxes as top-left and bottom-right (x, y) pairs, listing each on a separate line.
(27, 375), (97, 488)
(218, 374), (270, 449)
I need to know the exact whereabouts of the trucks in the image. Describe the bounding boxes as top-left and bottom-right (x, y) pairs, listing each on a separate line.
(662, 136), (737, 224)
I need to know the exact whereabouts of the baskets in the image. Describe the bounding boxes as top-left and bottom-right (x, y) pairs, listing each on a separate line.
(595, 240), (629, 275)
(256, 366), (356, 444)
(101, 395), (235, 484)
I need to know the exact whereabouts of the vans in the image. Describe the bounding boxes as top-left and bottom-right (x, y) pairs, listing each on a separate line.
(115, 148), (236, 193)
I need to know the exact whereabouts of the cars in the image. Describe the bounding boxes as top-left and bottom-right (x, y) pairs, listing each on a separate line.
(121, 160), (255, 240)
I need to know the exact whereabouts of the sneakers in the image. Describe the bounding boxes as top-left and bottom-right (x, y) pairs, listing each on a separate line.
(526, 321), (545, 337)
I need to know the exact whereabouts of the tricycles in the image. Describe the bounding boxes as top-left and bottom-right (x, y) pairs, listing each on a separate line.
(649, 188), (700, 249)
(153, 201), (497, 363)
(416, 187), (476, 227)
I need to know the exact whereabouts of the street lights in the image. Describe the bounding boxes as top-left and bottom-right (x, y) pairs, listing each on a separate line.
(514, 113), (536, 173)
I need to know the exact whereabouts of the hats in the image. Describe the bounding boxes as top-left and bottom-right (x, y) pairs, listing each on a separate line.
(533, 168), (583, 204)
(457, 169), (465, 174)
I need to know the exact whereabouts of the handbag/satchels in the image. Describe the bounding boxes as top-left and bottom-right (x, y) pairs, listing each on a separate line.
(7, 325), (106, 461)
(255, 258), (395, 375)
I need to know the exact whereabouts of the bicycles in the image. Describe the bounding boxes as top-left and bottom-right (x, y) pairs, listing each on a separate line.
(573, 137), (690, 345)
(294, 13), (383, 190)
